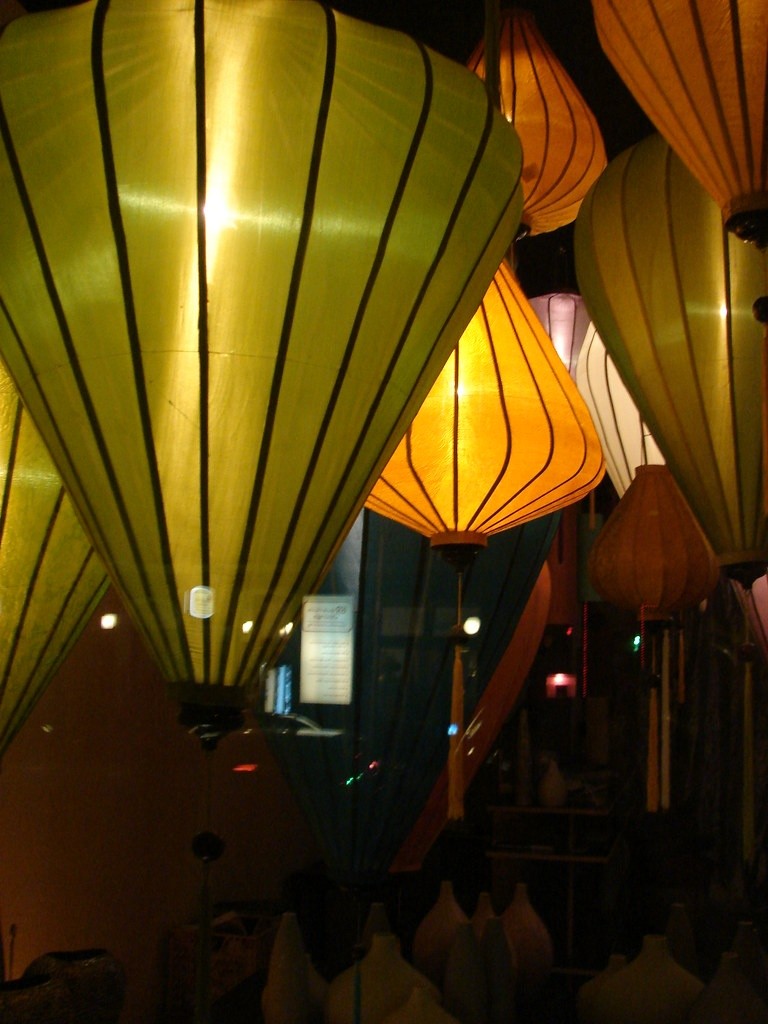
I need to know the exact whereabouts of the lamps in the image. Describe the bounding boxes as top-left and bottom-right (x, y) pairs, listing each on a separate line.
(0, 0), (767, 747)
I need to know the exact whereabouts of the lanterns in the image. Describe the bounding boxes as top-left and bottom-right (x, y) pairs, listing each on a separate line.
(1, 0), (767, 761)
